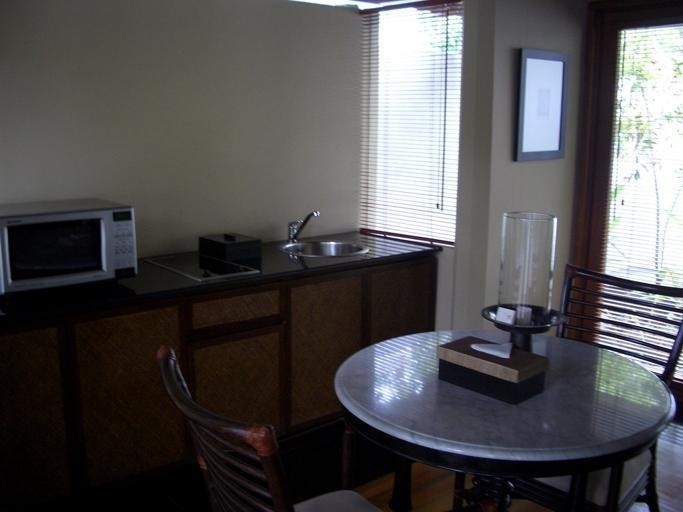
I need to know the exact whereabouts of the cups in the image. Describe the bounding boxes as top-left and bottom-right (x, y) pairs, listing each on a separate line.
(497, 212), (557, 325)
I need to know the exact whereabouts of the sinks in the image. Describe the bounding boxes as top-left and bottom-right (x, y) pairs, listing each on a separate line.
(282, 241), (370, 258)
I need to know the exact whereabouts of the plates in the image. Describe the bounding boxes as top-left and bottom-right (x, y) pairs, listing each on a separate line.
(481, 302), (569, 352)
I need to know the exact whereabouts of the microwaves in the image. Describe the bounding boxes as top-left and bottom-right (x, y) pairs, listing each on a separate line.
(0, 197), (139, 295)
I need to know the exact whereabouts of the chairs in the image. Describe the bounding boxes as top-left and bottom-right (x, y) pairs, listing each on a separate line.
(490, 262), (682, 512)
(156, 344), (383, 512)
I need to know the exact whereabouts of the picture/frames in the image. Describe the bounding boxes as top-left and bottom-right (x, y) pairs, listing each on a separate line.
(512, 46), (567, 165)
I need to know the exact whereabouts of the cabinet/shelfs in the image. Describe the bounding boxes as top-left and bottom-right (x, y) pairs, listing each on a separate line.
(0, 301), (185, 512)
(286, 257), (436, 436)
(186, 279), (286, 468)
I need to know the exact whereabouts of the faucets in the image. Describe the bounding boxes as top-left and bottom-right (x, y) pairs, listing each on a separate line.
(289, 211), (320, 244)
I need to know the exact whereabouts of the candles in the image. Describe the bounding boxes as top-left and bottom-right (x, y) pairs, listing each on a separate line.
(515, 306), (531, 327)
